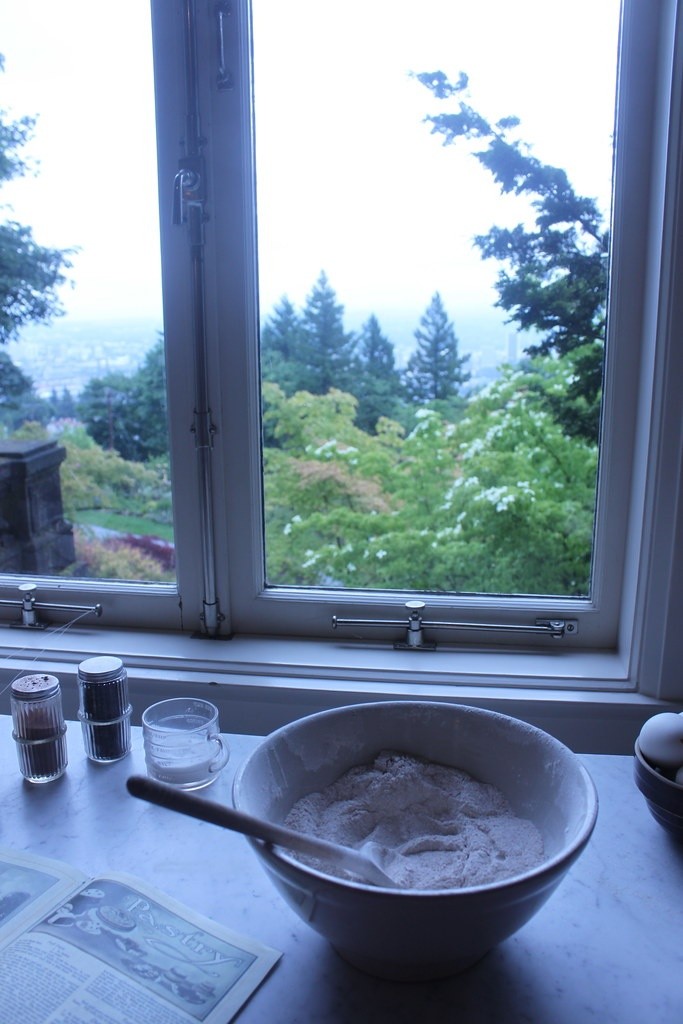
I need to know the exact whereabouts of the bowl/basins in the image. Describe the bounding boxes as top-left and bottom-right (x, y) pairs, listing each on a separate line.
(231, 701), (600, 984)
(633, 736), (683, 834)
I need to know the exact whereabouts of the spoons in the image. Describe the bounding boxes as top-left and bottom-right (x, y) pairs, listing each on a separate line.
(126, 774), (412, 890)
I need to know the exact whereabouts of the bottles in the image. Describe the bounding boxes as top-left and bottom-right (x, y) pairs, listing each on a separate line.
(11, 674), (68, 785)
(75, 656), (134, 763)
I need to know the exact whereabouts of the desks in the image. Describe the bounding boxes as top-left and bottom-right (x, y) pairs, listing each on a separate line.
(0, 716), (683, 1024)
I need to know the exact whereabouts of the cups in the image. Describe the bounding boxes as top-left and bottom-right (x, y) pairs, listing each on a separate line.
(142, 697), (231, 792)
